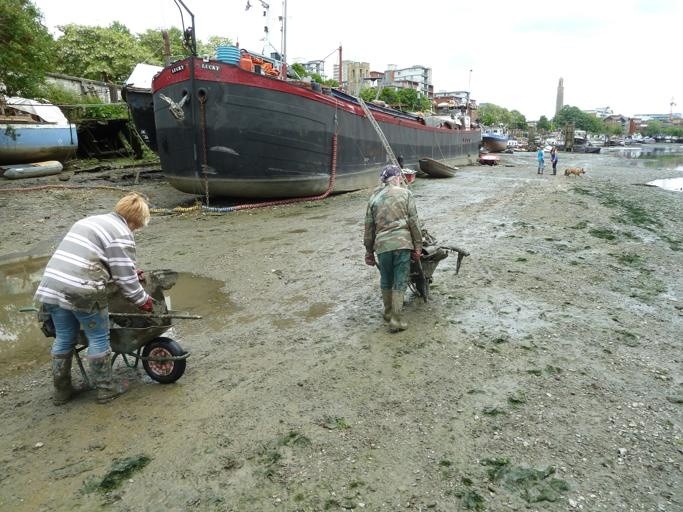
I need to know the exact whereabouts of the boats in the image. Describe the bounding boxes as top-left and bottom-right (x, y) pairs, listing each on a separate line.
(517, 127), (659, 155)
(0, 85), (86, 160)
(0, 160), (64, 180)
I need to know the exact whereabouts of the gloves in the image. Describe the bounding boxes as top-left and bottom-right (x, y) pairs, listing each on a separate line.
(134, 267), (146, 282)
(138, 294), (154, 313)
(365, 250), (376, 267)
(411, 248), (423, 262)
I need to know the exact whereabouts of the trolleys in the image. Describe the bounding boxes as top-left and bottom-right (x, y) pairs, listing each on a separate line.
(363, 237), (450, 299)
(16, 261), (193, 384)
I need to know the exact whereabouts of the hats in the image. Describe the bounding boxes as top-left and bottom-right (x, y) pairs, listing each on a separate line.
(380, 164), (403, 184)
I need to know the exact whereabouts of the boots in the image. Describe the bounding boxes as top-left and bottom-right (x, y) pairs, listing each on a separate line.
(549, 167), (557, 175)
(50, 350), (83, 407)
(389, 289), (409, 333)
(380, 287), (393, 322)
(537, 167), (544, 175)
(87, 346), (130, 405)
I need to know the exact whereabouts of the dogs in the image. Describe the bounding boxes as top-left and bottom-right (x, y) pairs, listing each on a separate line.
(564, 167), (586, 180)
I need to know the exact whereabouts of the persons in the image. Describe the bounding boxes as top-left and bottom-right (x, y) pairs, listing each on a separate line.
(32, 194), (155, 406)
(363, 164), (423, 333)
(550, 147), (558, 175)
(537, 145), (545, 174)
